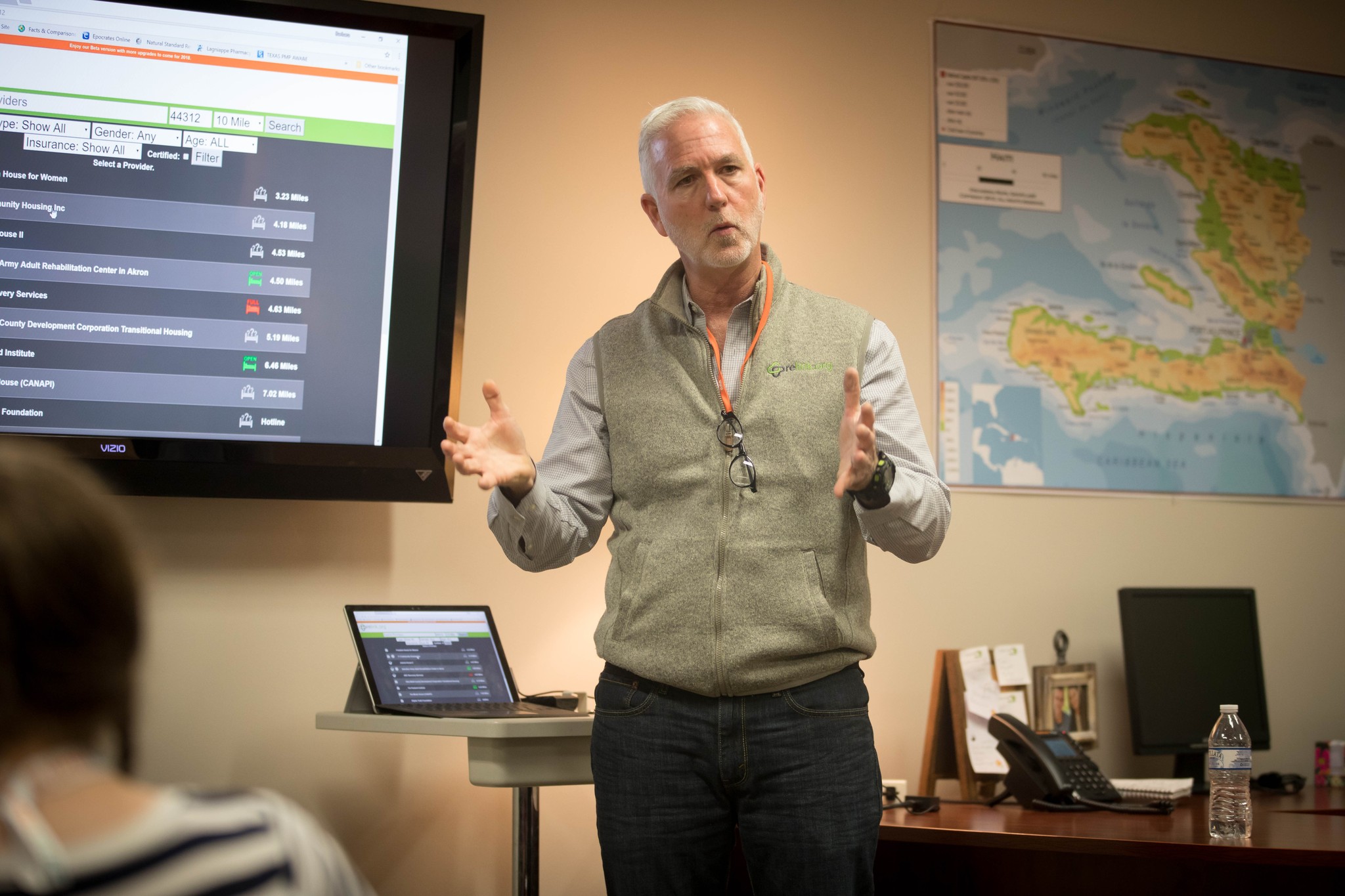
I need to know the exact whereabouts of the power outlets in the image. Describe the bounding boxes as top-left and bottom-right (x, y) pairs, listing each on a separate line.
(881, 779), (906, 805)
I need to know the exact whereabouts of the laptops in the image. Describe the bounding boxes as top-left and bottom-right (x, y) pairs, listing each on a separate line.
(343, 605), (587, 717)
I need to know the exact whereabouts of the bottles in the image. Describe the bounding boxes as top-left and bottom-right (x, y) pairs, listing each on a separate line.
(1207, 704), (1253, 842)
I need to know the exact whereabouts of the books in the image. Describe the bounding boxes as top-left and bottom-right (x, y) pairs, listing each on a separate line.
(1110, 778), (1194, 801)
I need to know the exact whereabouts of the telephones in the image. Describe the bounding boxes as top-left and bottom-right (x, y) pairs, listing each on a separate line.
(987, 712), (1122, 809)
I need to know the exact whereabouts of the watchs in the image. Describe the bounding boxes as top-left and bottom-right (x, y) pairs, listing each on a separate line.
(846, 450), (896, 499)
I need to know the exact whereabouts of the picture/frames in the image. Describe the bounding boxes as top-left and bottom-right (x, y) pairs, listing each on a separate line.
(1033, 663), (1096, 745)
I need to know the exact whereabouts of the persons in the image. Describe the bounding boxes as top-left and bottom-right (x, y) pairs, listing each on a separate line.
(0, 435), (375, 896)
(441, 96), (948, 896)
(1052, 686), (1089, 733)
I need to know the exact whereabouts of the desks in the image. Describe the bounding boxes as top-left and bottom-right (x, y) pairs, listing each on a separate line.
(316, 713), (595, 896)
(878, 783), (1345, 896)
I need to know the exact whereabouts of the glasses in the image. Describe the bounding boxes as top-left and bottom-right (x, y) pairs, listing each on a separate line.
(717, 411), (758, 494)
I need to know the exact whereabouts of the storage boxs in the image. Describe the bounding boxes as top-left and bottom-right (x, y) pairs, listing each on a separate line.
(1315, 739), (1345, 788)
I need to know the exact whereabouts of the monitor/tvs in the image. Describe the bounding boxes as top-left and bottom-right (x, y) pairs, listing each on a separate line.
(1119, 587), (1271, 794)
(0, 0), (486, 500)
